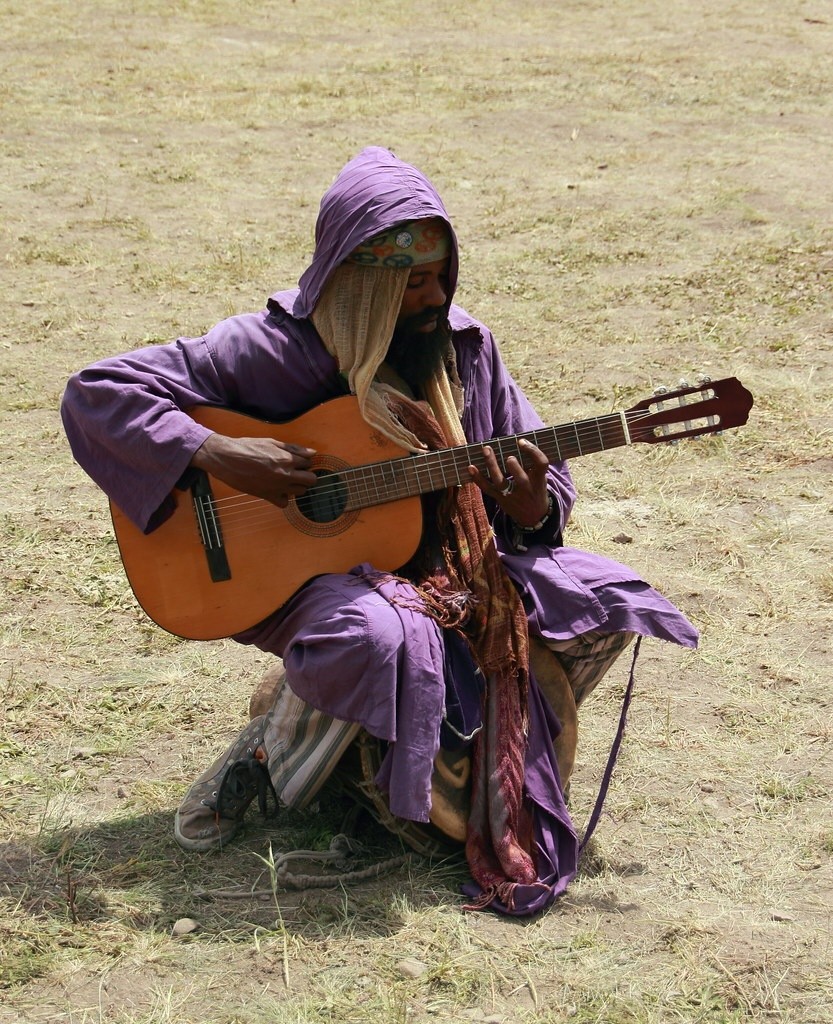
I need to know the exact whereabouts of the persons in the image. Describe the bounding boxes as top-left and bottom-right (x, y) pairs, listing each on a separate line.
(60, 146), (697, 918)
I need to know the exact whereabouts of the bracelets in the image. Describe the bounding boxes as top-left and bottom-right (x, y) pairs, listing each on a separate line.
(512, 498), (554, 531)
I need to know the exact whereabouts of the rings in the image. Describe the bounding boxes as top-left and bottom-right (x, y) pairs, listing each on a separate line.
(501, 481), (513, 496)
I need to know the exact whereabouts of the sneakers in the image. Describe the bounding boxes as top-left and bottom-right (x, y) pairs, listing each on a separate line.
(395, 812), (463, 863)
(172, 714), (283, 854)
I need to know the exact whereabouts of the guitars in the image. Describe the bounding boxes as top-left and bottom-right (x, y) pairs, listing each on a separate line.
(110, 376), (755, 638)
